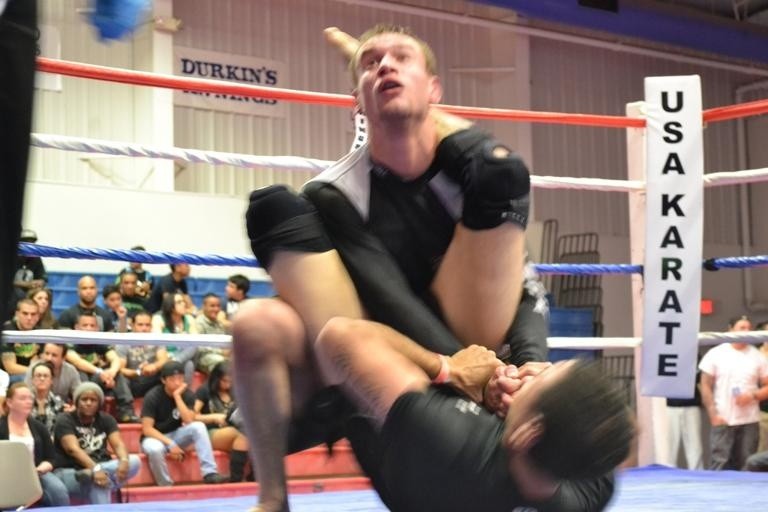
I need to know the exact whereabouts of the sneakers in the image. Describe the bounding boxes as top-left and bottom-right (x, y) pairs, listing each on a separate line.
(205, 472), (222, 483)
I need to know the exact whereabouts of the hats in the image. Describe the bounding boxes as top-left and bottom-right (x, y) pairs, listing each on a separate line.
(72, 380), (105, 410)
(161, 361), (184, 378)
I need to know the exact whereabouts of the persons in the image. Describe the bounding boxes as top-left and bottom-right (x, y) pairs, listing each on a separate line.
(249, 23), (638, 509)
(0, 242), (251, 511)
(653, 352), (705, 472)
(752, 321), (768, 454)
(229, 24), (550, 510)
(745, 448), (768, 470)
(698, 314), (768, 472)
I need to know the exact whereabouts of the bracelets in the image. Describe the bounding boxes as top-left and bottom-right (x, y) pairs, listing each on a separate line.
(433, 351), (451, 385)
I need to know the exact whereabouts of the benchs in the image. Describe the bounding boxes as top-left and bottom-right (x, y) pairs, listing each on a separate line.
(65, 362), (374, 507)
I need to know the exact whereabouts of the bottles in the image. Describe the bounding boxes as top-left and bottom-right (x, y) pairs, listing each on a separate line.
(730, 386), (744, 408)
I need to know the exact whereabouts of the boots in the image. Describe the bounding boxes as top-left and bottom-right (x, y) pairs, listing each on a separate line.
(230, 450), (245, 482)
